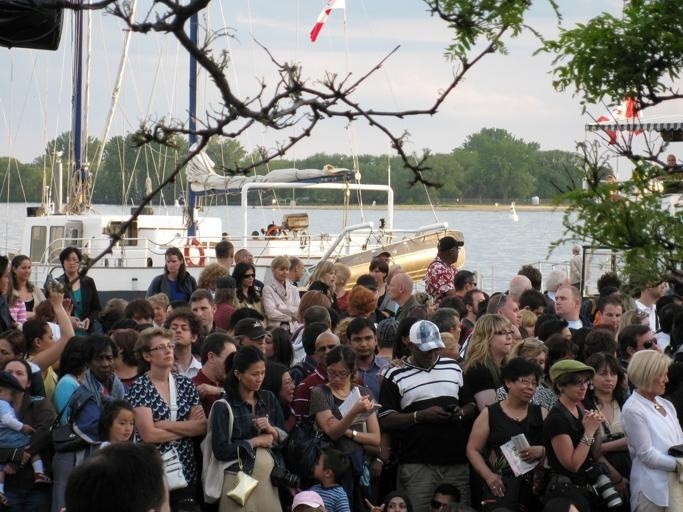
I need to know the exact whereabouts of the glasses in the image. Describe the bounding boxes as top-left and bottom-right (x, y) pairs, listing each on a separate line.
(325, 371), (350, 379)
(644, 338), (657, 348)
(242, 274), (255, 278)
(430, 499), (447, 509)
(146, 343), (176, 351)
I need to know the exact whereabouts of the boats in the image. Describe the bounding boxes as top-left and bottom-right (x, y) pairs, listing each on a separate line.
(526, 91), (683, 312)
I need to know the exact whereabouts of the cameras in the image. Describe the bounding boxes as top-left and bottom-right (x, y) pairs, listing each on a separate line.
(270, 465), (300, 488)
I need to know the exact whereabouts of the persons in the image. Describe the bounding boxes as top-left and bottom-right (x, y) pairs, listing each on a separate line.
(662, 155), (682, 174)
(0, 223), (681, 512)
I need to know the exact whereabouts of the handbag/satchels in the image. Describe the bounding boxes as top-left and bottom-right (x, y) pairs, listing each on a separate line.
(200, 398), (240, 504)
(282, 424), (320, 472)
(49, 424), (88, 452)
(227, 470), (259, 507)
(159, 446), (188, 492)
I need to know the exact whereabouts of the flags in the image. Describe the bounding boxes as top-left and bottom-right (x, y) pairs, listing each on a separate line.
(596, 97), (643, 144)
(310, 0), (344, 43)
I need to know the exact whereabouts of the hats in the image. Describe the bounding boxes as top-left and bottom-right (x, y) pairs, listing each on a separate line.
(0, 371), (25, 392)
(549, 359), (596, 383)
(409, 319), (446, 351)
(376, 319), (400, 341)
(234, 318), (272, 339)
(438, 237), (464, 251)
(291, 490), (325, 512)
(373, 247), (391, 258)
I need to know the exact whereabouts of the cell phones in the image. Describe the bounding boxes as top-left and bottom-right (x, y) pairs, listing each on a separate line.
(445, 404), (456, 413)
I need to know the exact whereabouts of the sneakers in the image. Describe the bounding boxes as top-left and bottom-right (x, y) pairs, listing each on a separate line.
(0, 493), (11, 507)
(34, 473), (54, 484)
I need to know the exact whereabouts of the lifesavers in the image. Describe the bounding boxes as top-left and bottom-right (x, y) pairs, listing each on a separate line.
(184, 239), (205, 266)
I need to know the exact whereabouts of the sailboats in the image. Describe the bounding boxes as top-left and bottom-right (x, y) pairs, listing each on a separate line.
(0, 0), (466, 308)
